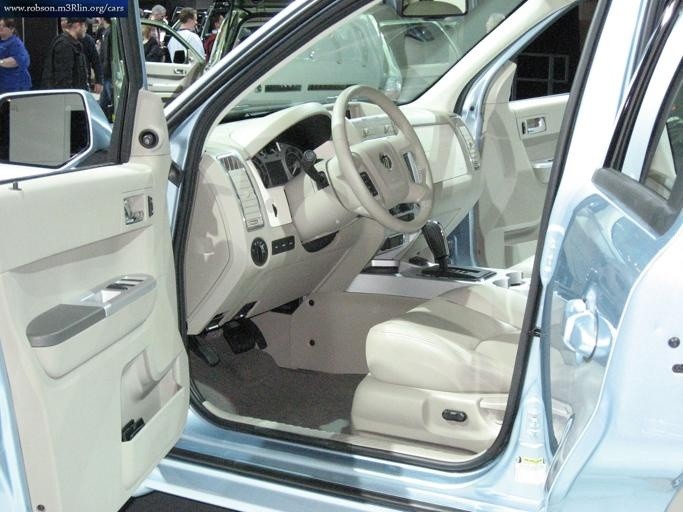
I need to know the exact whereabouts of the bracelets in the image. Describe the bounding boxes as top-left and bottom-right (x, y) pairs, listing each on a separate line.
(0, 59), (4, 65)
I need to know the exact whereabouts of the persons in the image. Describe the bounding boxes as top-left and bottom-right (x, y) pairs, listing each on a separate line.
(38, 0), (228, 121)
(0, 13), (34, 99)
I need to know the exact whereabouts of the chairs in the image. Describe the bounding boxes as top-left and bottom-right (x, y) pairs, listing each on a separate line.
(349, 254), (620, 456)
